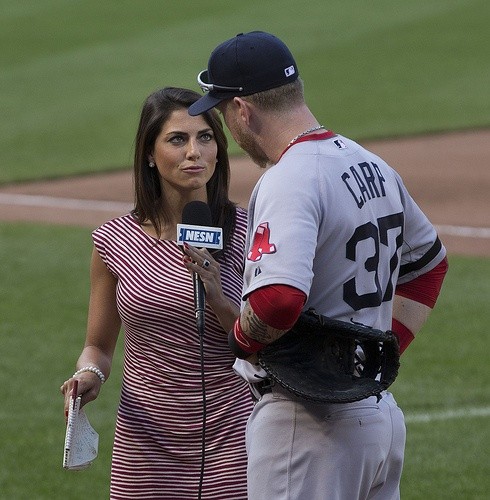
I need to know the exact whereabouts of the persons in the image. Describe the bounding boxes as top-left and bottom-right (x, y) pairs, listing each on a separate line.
(61, 86), (257, 500)
(188, 31), (448, 500)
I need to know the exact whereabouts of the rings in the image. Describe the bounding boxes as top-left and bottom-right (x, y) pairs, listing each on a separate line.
(202, 260), (210, 269)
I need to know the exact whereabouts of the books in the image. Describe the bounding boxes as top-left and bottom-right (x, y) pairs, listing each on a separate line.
(63, 396), (99, 471)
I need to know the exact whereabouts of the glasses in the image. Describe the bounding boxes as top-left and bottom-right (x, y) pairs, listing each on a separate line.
(197, 68), (243, 93)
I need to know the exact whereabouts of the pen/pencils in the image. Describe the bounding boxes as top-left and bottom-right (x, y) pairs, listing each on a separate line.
(72, 380), (79, 410)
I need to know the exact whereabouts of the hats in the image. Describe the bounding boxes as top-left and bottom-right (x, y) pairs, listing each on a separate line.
(187, 31), (299, 116)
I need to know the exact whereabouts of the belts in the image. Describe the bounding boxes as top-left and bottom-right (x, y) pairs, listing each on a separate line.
(250, 379), (271, 402)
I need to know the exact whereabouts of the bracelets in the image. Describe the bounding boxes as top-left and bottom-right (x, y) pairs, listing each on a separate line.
(72, 367), (105, 385)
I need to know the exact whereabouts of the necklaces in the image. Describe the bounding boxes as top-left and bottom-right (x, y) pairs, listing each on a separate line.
(285, 125), (324, 147)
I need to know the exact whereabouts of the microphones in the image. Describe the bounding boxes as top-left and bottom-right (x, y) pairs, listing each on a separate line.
(176, 201), (223, 336)
